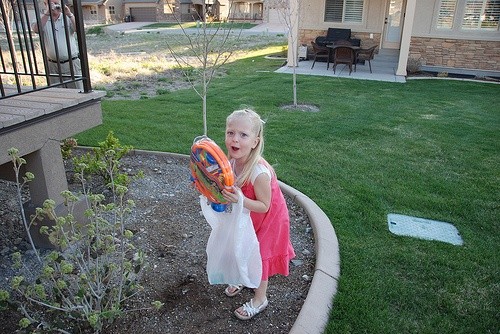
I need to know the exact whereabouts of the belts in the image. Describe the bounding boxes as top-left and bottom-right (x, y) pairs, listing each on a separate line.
(48, 57), (77, 64)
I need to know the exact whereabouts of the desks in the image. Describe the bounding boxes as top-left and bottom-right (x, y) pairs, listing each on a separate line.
(317, 38), (361, 65)
(326, 45), (361, 71)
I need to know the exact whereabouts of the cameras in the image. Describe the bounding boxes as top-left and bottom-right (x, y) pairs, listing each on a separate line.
(52, 5), (61, 11)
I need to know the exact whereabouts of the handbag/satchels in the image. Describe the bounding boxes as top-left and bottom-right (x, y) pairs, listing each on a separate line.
(199, 190), (263, 288)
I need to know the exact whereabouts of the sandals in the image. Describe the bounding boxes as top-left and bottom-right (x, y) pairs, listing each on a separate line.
(224, 284), (244, 297)
(234, 297), (268, 320)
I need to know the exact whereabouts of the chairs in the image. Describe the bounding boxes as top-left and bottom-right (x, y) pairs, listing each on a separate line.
(310, 41), (330, 71)
(354, 44), (378, 74)
(332, 45), (354, 76)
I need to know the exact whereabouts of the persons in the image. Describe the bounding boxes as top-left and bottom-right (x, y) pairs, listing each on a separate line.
(33, 0), (84, 89)
(199, 110), (296, 320)
